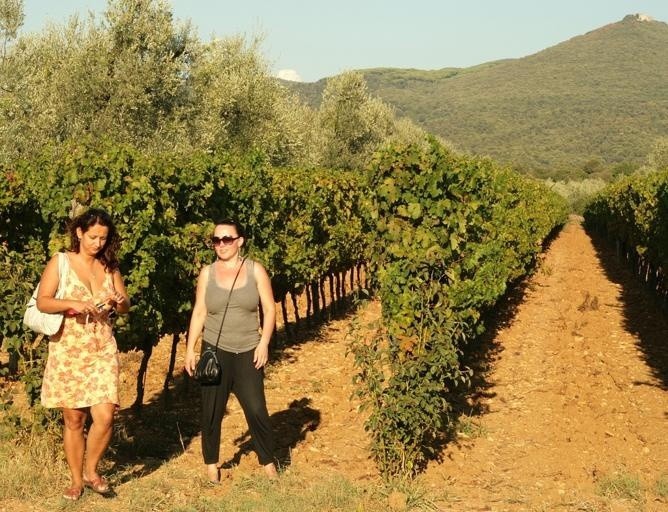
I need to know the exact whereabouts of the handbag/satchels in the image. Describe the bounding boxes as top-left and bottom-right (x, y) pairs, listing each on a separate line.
(193, 350), (221, 384)
(23, 252), (69, 336)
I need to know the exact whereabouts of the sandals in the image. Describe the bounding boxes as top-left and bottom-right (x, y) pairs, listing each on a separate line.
(63, 472), (110, 500)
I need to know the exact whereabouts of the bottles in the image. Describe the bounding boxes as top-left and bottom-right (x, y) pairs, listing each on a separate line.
(93, 298), (113, 322)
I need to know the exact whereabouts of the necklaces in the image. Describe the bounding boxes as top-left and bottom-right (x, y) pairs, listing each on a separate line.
(73, 252), (103, 281)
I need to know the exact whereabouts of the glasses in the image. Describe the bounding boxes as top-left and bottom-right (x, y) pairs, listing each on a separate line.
(212, 235), (240, 246)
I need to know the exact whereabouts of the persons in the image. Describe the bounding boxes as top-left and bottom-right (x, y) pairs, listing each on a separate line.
(23, 208), (131, 501)
(184, 219), (280, 490)
